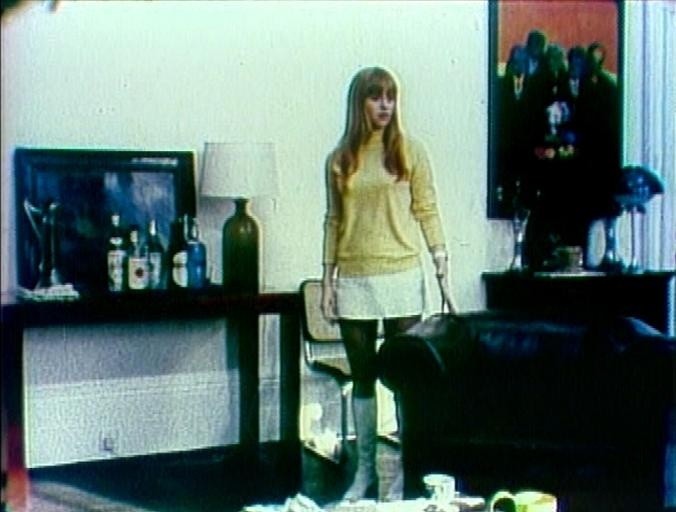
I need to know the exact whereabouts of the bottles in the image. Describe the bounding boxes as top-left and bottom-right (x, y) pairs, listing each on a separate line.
(105, 212), (208, 292)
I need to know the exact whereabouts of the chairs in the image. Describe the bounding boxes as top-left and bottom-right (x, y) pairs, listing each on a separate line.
(300, 279), (402, 511)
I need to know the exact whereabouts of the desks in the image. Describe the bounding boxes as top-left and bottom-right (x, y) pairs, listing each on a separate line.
(0, 290), (305, 511)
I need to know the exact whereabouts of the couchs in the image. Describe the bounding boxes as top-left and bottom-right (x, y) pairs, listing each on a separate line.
(376, 311), (675, 510)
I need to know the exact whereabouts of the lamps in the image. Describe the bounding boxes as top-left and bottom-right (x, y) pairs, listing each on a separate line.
(198, 139), (273, 290)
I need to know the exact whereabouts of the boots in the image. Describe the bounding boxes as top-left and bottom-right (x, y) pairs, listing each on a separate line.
(342, 397), (380, 503)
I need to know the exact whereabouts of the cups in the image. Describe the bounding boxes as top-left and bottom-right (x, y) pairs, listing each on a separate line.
(482, 489), (558, 512)
(422, 473), (457, 512)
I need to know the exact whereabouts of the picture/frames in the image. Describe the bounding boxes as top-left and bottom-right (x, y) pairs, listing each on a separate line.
(12, 147), (195, 288)
(484, 0), (626, 220)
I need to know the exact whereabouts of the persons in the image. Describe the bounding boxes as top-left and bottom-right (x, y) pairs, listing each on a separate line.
(517, 164), (666, 272)
(318, 65), (461, 503)
(489, 27), (620, 213)
